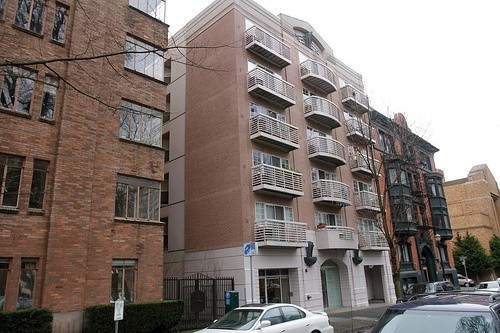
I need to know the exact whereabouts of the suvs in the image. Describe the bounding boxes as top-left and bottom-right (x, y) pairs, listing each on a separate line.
(396, 281), (459, 315)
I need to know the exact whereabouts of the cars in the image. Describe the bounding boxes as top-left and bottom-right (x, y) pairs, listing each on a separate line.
(458, 275), (475, 287)
(474, 280), (500, 293)
(192, 301), (334, 333)
(360, 296), (500, 333)
